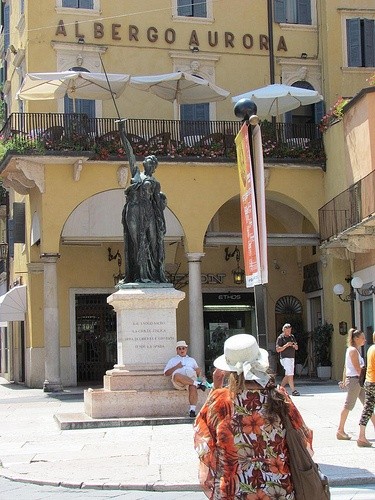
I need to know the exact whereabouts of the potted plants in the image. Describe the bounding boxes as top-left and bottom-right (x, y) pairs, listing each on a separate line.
(310, 321), (334, 381)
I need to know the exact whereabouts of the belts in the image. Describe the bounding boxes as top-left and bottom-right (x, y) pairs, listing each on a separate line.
(347, 376), (360, 378)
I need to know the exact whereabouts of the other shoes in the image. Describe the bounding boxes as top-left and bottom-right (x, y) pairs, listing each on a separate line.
(190, 410), (196, 417)
(292, 390), (300, 396)
(197, 382), (206, 391)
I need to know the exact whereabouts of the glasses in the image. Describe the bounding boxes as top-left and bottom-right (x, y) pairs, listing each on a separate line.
(179, 347), (187, 350)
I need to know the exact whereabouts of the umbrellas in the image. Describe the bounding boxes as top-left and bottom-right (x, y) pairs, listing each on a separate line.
(16, 73), (130, 113)
(231, 82), (326, 142)
(130, 70), (230, 139)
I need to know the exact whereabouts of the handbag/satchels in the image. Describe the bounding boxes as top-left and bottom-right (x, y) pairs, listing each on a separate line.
(279, 410), (331, 500)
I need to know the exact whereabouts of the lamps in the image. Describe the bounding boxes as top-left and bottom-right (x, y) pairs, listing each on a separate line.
(108, 248), (124, 285)
(301, 52), (308, 59)
(225, 246), (245, 285)
(193, 46), (199, 52)
(78, 39), (85, 44)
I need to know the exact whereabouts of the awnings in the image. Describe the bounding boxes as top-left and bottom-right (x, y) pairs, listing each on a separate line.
(0, 277), (27, 327)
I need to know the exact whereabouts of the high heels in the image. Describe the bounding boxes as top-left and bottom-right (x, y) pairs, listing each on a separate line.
(356, 438), (373, 447)
(337, 431), (351, 440)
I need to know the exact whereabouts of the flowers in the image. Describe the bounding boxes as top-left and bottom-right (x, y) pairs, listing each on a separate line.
(365, 73), (375, 87)
(0, 119), (326, 170)
(318, 96), (353, 134)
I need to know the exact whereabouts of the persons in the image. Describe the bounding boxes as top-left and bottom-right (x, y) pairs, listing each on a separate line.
(194, 333), (314, 500)
(276, 323), (300, 395)
(118, 121), (167, 283)
(164, 341), (206, 418)
(357, 330), (375, 448)
(335, 328), (375, 440)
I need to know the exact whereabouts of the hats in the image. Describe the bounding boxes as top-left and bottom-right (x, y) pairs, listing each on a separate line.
(175, 340), (189, 348)
(213, 333), (272, 389)
